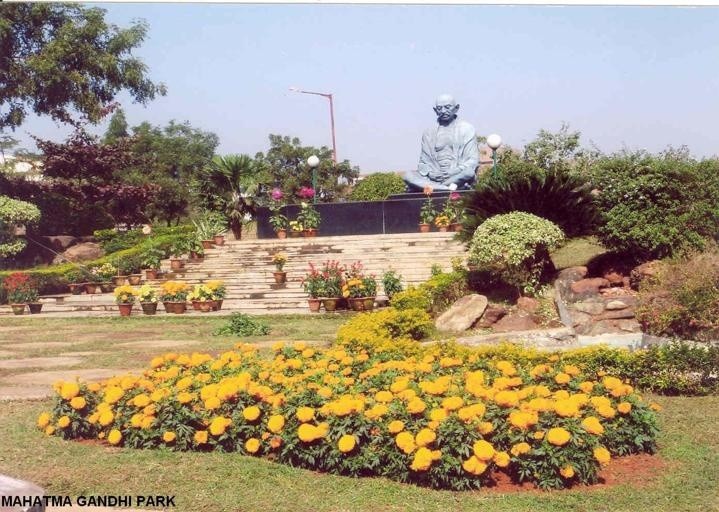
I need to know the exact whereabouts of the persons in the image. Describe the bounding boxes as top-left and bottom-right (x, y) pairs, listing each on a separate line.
(401, 93), (481, 190)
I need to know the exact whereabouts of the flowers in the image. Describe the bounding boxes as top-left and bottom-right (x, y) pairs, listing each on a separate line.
(418, 183), (468, 227)
(3, 269), (56, 305)
(265, 183), (324, 232)
(112, 275), (228, 301)
(270, 251), (287, 272)
(297, 257), (381, 299)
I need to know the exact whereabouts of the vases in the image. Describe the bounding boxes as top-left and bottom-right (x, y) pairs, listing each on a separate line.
(119, 298), (224, 317)
(275, 227), (320, 239)
(10, 303), (44, 315)
(273, 272), (287, 283)
(419, 223), (465, 232)
(306, 298), (380, 311)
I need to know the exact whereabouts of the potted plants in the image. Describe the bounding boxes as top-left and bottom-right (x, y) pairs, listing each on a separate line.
(62, 210), (226, 294)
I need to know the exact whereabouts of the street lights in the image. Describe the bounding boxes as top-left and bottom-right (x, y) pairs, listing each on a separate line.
(488, 134), (501, 183)
(308, 155), (319, 203)
(288, 86), (336, 165)
